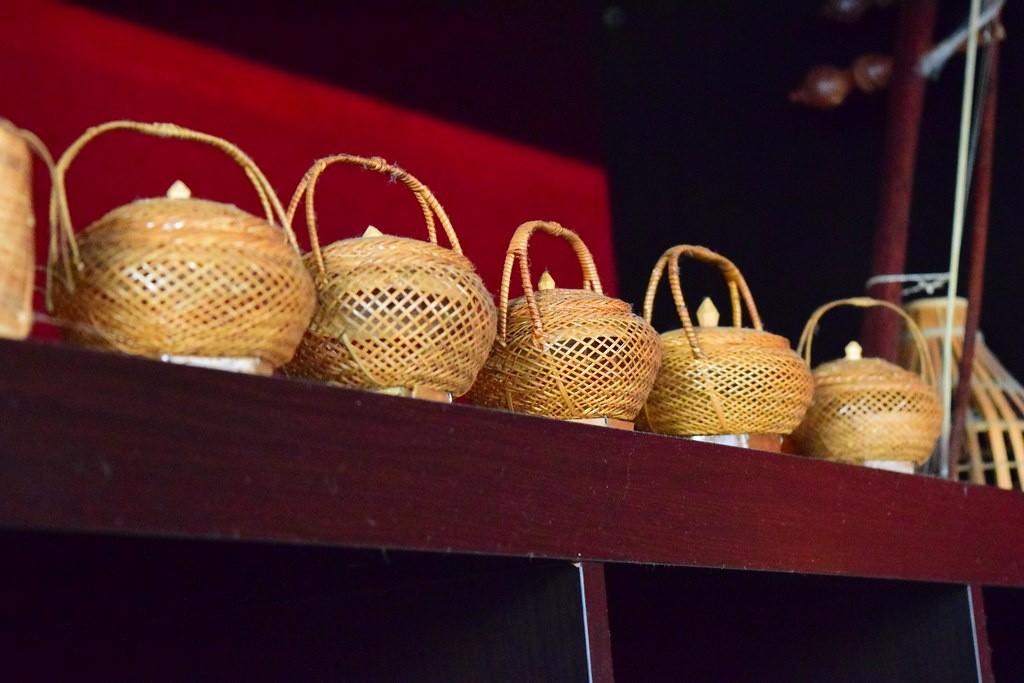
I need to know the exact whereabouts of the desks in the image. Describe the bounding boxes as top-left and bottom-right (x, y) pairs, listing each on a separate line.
(0, 337), (1024, 683)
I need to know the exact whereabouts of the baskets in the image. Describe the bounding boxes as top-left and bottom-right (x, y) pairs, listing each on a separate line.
(0, 117), (37, 343)
(797, 296), (944, 469)
(469, 220), (662, 430)
(284, 152), (499, 404)
(46, 118), (317, 378)
(642, 244), (814, 454)
